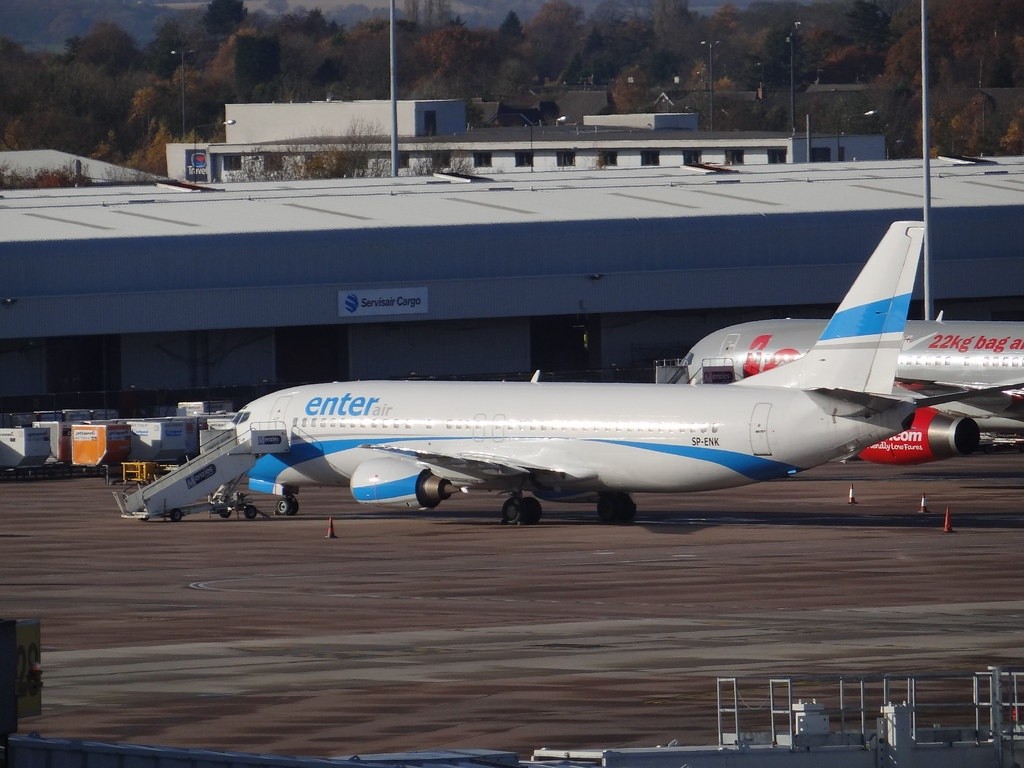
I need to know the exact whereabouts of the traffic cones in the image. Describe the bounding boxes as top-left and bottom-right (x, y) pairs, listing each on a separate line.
(941, 506), (958, 535)
(323, 515), (340, 539)
(917, 491), (931, 515)
(845, 482), (858, 504)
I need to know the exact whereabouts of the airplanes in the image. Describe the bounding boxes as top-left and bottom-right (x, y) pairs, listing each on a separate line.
(652, 308), (1024, 466)
(109, 215), (1024, 528)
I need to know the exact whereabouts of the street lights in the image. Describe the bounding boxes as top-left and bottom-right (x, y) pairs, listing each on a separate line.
(170, 50), (197, 143)
(785, 22), (803, 135)
(530, 114), (568, 172)
(192, 119), (237, 184)
(700, 39), (722, 132)
(837, 109), (880, 161)
